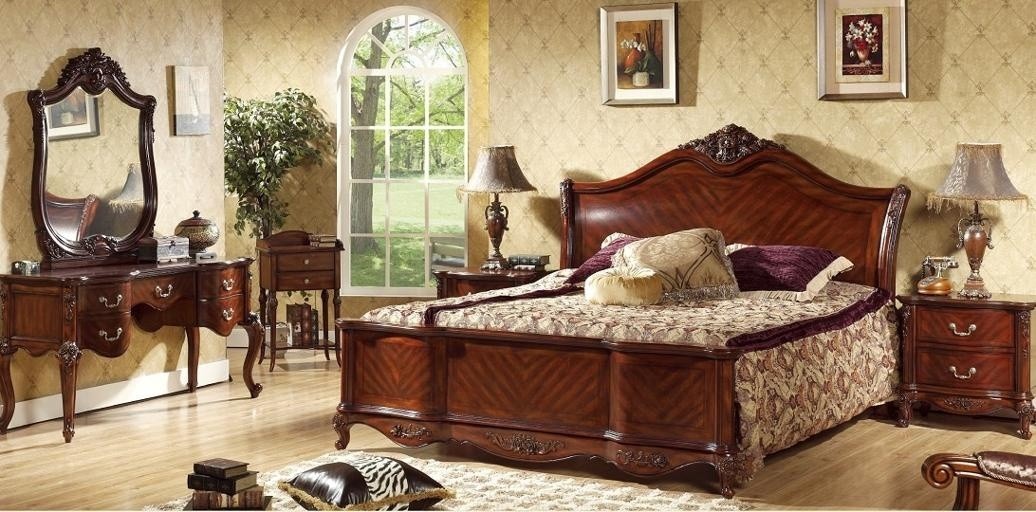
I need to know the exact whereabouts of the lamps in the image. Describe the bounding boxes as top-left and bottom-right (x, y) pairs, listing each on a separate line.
(455, 146), (538, 272)
(926, 142), (1030, 300)
(107, 161), (145, 214)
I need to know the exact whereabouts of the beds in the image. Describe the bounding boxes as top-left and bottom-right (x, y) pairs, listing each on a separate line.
(45, 192), (102, 243)
(333, 123), (911, 500)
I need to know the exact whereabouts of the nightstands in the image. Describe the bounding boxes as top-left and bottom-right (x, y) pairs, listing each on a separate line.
(432, 260), (546, 299)
(894, 290), (1036, 440)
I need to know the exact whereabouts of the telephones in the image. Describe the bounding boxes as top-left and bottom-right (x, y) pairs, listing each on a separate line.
(917, 255), (959, 295)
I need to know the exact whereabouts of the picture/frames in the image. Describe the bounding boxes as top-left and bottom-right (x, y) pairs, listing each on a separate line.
(43, 86), (100, 142)
(815, 1), (909, 102)
(599, 2), (679, 107)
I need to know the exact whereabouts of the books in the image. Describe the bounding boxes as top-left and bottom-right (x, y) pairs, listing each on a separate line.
(183, 458), (273, 511)
(286, 302), (319, 349)
(508, 254), (551, 272)
(307, 234), (337, 248)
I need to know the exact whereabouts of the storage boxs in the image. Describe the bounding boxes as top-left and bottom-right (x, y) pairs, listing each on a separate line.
(152, 234), (190, 262)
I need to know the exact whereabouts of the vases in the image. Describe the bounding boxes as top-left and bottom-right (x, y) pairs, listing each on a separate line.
(625, 32), (645, 76)
(855, 44), (870, 65)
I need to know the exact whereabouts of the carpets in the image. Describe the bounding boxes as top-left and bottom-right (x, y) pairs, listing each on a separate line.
(140, 451), (753, 510)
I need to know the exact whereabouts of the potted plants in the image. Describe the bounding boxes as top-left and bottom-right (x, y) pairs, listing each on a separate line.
(223, 87), (337, 358)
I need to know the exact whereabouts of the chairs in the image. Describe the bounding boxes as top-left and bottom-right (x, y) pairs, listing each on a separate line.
(920, 450), (1032, 508)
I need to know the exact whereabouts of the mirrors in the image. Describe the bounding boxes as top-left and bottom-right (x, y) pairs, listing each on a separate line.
(27, 46), (158, 273)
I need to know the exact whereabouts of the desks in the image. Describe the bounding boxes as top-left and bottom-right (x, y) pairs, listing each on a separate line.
(255, 229), (345, 372)
(0, 257), (266, 441)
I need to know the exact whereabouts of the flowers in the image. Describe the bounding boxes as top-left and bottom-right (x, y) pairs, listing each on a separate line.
(845, 19), (880, 65)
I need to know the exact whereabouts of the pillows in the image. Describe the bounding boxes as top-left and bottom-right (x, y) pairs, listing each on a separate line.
(276, 451), (455, 511)
(610, 228), (740, 304)
(724, 242), (855, 303)
(561, 231), (644, 289)
